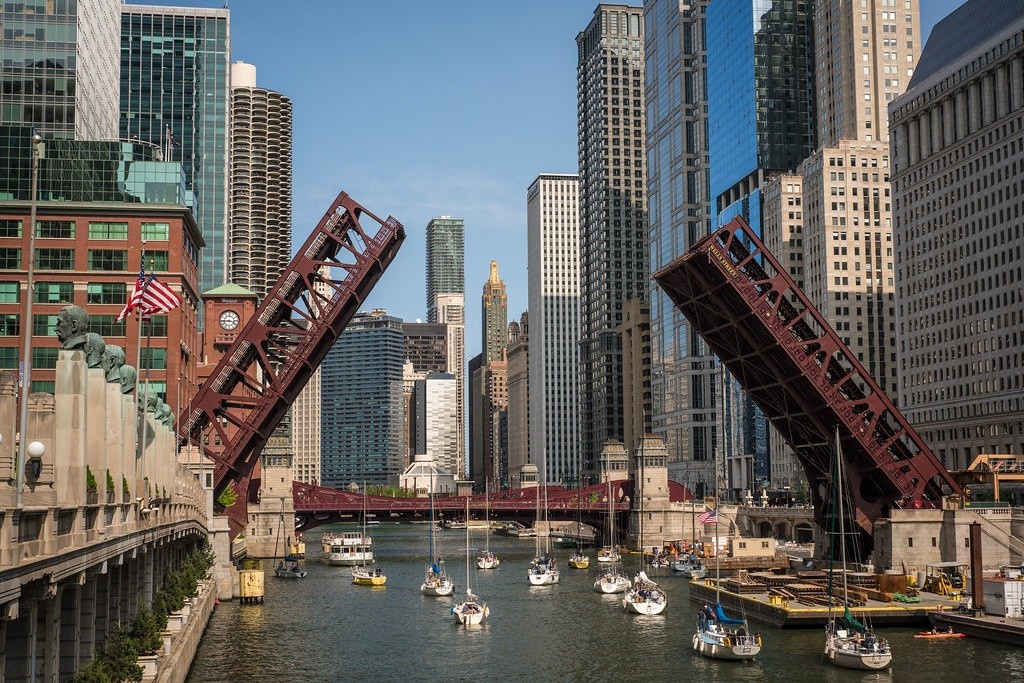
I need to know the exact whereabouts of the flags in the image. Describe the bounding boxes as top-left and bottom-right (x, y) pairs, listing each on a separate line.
(111, 254), (147, 325)
(135, 272), (184, 323)
(697, 510), (717, 525)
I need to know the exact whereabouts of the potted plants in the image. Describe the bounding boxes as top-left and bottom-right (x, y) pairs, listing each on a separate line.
(67, 532), (217, 683)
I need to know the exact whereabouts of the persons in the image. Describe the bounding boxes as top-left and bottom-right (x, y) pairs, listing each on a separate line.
(429, 572), (436, 586)
(278, 559), (300, 572)
(530, 547), (588, 578)
(627, 586), (661, 604)
(833, 624), (889, 657)
(376, 566), (381, 577)
(703, 605), (710, 631)
(946, 625), (954, 635)
(697, 608), (706, 633)
(737, 625), (747, 645)
(52, 305), (177, 432)
(651, 536), (704, 568)
(931, 626), (939, 635)
(461, 602), (478, 614)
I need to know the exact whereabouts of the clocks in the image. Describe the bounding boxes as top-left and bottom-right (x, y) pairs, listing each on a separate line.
(219, 310), (240, 331)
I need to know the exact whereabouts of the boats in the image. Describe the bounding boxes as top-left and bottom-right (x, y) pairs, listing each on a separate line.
(914, 631), (966, 639)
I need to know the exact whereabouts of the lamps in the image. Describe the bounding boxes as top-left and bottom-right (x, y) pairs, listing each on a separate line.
(28, 441), (45, 478)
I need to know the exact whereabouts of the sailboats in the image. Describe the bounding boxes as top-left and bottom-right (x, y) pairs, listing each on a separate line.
(273, 409), (893, 672)
